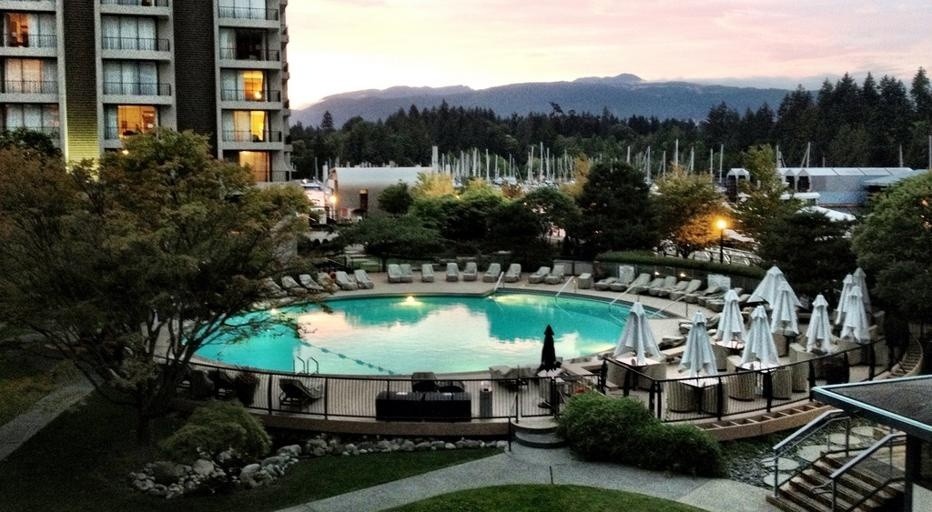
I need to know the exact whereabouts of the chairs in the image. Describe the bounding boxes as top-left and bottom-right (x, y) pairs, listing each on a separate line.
(172, 261), (886, 416)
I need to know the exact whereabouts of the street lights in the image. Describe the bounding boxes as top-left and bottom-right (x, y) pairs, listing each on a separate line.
(715, 218), (728, 265)
(329, 194), (337, 226)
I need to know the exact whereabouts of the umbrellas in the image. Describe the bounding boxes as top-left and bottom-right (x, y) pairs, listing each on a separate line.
(769, 280), (800, 337)
(612, 300), (662, 366)
(838, 285), (872, 345)
(715, 289), (746, 345)
(804, 293), (833, 353)
(740, 304), (783, 368)
(745, 265), (802, 312)
(676, 309), (719, 384)
(847, 266), (873, 315)
(835, 271), (857, 329)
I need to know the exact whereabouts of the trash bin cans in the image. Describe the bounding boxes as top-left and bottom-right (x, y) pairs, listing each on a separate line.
(479, 387), (493, 417)
